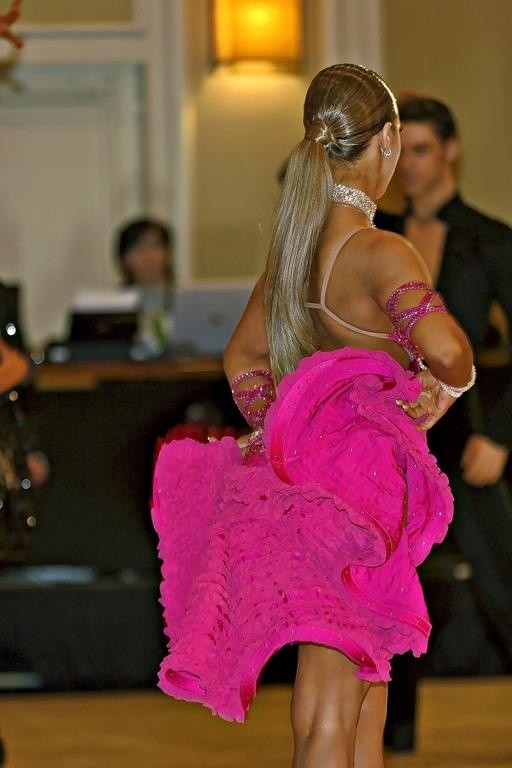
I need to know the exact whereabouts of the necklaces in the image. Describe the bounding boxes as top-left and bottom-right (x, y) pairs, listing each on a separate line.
(331, 181), (379, 225)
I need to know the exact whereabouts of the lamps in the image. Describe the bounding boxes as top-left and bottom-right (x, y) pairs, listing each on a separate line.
(209, 1), (307, 65)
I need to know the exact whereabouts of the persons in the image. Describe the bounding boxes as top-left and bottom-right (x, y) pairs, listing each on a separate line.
(104, 214), (181, 351)
(366, 90), (511, 756)
(205, 58), (477, 767)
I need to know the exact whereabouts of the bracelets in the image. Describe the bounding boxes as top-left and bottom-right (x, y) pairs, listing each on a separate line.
(434, 366), (478, 398)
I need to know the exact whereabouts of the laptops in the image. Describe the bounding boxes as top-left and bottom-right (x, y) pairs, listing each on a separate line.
(168, 287), (255, 358)
